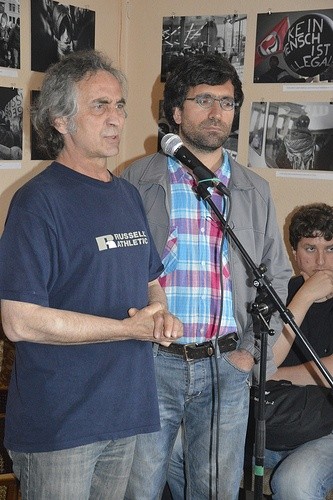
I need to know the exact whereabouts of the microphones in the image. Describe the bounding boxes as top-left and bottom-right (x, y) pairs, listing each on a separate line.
(160, 133), (232, 196)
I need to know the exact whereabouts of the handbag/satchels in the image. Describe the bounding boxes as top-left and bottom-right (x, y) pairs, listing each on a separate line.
(252, 379), (332, 452)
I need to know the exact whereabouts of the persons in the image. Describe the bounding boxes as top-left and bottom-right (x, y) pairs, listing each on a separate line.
(118, 50), (292, 500)
(50, 3), (78, 61)
(0, 50), (184, 500)
(254, 55), (332, 172)
(162, 40), (244, 84)
(0, 11), (23, 161)
(166, 202), (333, 500)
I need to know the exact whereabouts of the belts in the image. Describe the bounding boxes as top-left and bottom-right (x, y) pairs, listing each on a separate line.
(152, 333), (239, 360)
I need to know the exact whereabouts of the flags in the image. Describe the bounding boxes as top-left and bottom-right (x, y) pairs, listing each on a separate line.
(254, 15), (290, 68)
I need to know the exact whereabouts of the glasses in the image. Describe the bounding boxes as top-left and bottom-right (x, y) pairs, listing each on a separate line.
(183, 93), (240, 111)
